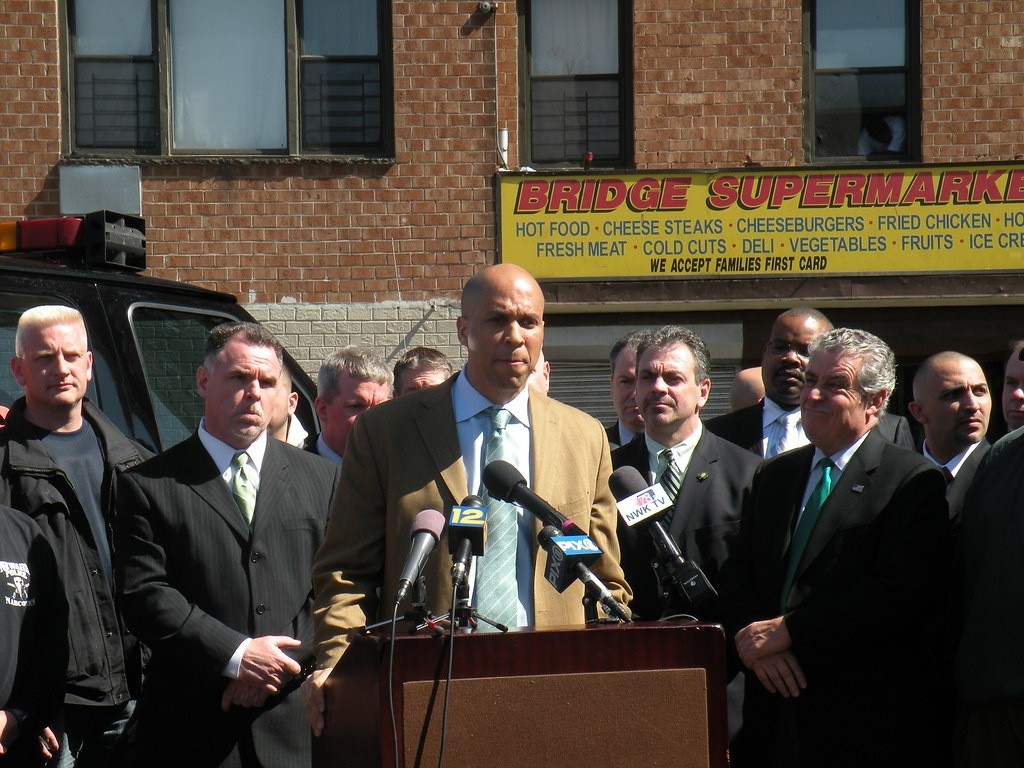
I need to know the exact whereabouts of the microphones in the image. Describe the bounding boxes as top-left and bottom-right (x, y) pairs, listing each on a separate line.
(393, 508), (447, 605)
(482, 461), (588, 536)
(608, 467), (686, 568)
(448, 494), (489, 587)
(536, 524), (631, 622)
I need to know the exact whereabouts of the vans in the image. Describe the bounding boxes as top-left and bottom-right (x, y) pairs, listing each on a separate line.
(1, 211), (322, 453)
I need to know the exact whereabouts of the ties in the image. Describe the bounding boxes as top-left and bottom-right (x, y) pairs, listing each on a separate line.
(657, 449), (682, 532)
(233, 452), (259, 534)
(775, 410), (802, 454)
(765, 458), (835, 620)
(941, 467), (954, 487)
(473, 408), (519, 633)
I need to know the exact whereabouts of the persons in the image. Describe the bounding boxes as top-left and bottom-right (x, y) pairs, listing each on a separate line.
(0, 304), (154, 768)
(267, 259), (629, 737)
(601, 304), (1024, 768)
(106, 325), (344, 768)
(857, 118), (905, 157)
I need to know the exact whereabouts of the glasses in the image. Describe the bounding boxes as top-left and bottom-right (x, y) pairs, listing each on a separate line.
(769, 339), (813, 357)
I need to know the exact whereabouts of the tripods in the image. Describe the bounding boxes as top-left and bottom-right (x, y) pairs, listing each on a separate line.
(407, 575), (508, 634)
(357, 577), (460, 636)
